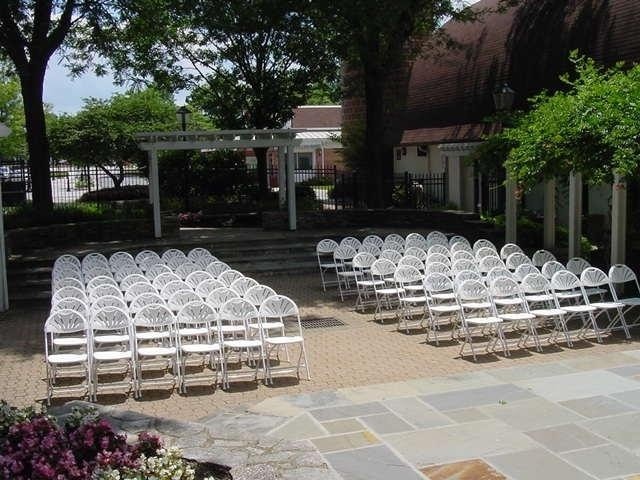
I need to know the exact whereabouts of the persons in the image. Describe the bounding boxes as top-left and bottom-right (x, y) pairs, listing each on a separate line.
(520, 183), (524, 219)
(514, 184), (521, 217)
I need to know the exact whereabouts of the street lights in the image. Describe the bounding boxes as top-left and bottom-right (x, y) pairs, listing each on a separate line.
(175, 105), (194, 155)
